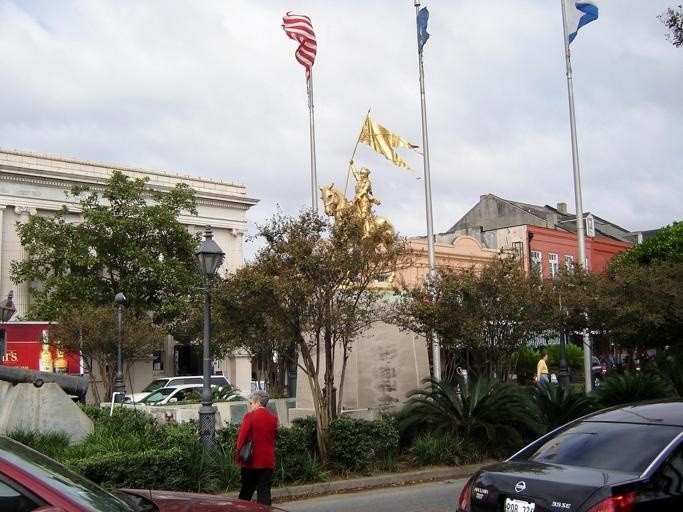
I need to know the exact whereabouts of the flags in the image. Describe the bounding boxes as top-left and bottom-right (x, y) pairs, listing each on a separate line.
(416, 6), (430, 54)
(280, 10), (318, 82)
(565, 0), (598, 45)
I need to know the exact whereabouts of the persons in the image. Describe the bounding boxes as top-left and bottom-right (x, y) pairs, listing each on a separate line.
(536, 353), (550, 382)
(350, 159), (373, 241)
(235, 390), (278, 506)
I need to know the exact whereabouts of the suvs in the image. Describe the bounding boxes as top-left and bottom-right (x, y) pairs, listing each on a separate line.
(569, 353), (604, 389)
(121, 373), (236, 406)
(599, 354), (636, 378)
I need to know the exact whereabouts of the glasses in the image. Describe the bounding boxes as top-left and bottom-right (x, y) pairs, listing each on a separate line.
(250, 401), (254, 405)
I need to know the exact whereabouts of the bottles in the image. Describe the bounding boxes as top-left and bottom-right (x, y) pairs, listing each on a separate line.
(38, 330), (69, 374)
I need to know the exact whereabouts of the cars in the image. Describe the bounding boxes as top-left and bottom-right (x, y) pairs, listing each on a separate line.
(127, 384), (234, 405)
(1, 431), (293, 512)
(456, 397), (682, 512)
(249, 381), (265, 393)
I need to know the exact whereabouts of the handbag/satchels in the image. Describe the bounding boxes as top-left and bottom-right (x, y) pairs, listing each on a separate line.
(240, 440), (254, 462)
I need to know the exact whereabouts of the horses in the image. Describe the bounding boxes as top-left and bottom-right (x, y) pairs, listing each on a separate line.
(317, 182), (397, 284)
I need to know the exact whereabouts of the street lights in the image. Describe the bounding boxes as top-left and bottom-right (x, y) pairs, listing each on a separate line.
(111, 292), (127, 407)
(0, 289), (20, 372)
(192, 223), (224, 492)
